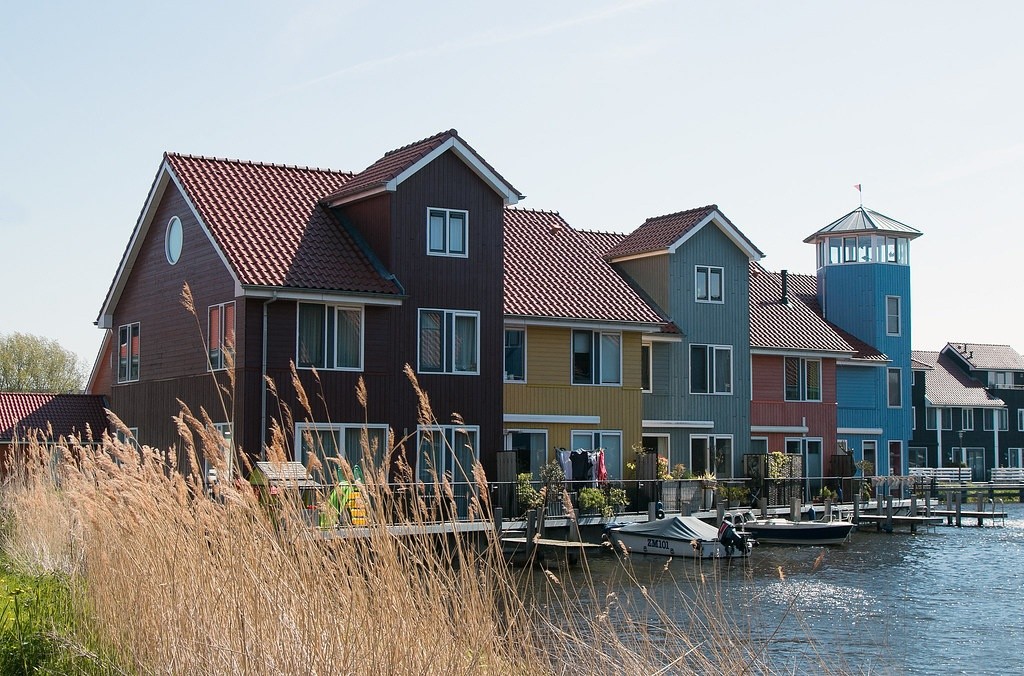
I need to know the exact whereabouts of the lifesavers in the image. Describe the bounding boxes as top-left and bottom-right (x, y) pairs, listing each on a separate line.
(657, 509), (665, 519)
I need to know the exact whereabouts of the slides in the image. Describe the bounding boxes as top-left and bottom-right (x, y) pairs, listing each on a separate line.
(320, 481), (354, 526)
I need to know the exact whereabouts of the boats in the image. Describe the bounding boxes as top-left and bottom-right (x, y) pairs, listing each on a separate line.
(733, 515), (858, 546)
(602, 516), (759, 558)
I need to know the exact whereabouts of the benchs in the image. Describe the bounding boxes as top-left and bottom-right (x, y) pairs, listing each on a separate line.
(909, 468), (973, 483)
(991, 467), (1024, 484)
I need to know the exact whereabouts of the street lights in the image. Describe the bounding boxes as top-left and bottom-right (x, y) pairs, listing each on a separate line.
(956, 430), (967, 480)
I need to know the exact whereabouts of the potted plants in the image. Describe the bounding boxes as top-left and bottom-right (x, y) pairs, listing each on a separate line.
(819, 487), (838, 503)
(699, 471), (751, 508)
